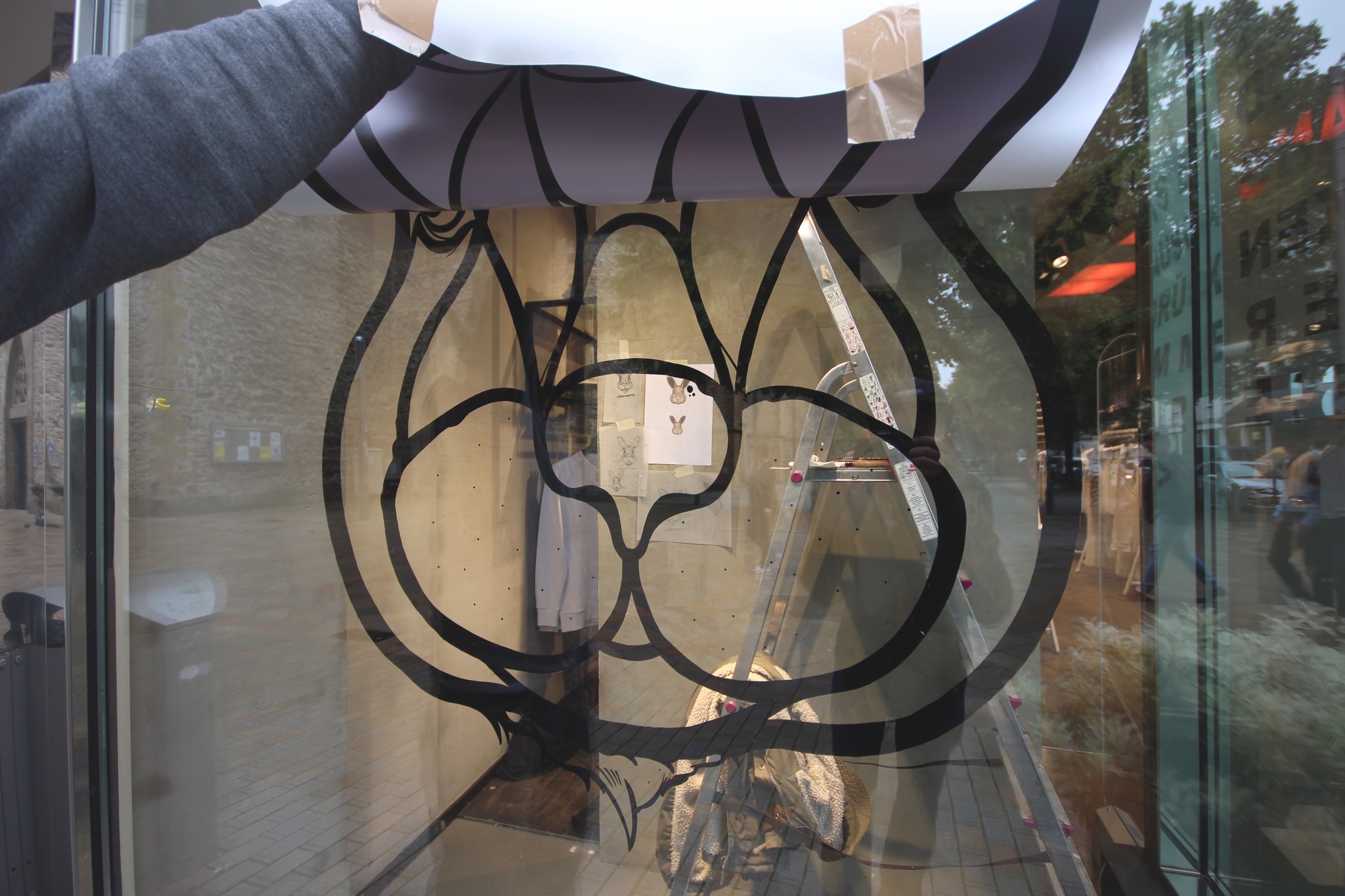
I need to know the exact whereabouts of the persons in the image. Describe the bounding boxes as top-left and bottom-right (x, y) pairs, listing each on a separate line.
(0, 1), (421, 352)
(1122, 422), (1345, 609)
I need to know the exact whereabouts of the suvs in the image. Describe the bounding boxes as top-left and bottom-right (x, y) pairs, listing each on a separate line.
(1194, 458), (1288, 523)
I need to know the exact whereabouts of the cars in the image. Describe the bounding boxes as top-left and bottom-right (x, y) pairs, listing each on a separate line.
(1020, 445), (1150, 510)
